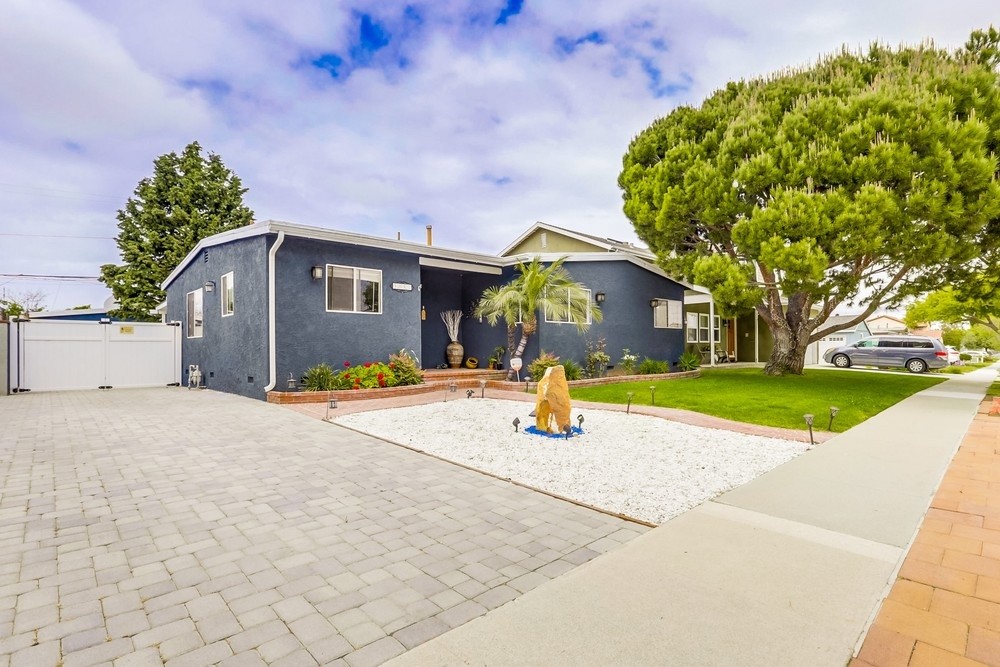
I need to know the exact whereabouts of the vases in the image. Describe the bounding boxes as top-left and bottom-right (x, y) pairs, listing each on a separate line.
(446, 339), (464, 369)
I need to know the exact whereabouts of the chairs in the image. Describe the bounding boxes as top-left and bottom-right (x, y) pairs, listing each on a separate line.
(696, 344), (711, 364)
(714, 343), (736, 363)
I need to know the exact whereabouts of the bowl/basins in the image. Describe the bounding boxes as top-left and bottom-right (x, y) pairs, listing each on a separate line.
(466, 357), (478, 369)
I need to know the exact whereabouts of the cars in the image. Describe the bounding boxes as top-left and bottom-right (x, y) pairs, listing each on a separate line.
(944, 346), (961, 365)
(823, 336), (949, 373)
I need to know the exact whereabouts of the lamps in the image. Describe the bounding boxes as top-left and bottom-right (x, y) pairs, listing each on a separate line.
(803, 413), (816, 426)
(561, 423), (572, 440)
(744, 331), (749, 338)
(723, 319), (727, 328)
(626, 392), (636, 402)
(479, 379), (487, 389)
(328, 393), (338, 409)
(312, 264), (324, 281)
(649, 298), (659, 311)
(595, 291), (605, 306)
(512, 416), (521, 433)
(449, 383), (458, 393)
(287, 374), (297, 389)
(577, 413), (585, 428)
(205, 279), (216, 292)
(649, 385), (657, 395)
(829, 406), (841, 418)
(524, 375), (531, 383)
